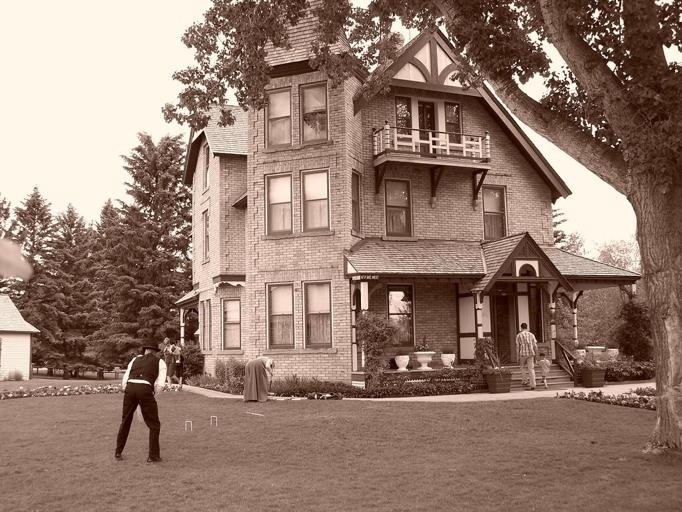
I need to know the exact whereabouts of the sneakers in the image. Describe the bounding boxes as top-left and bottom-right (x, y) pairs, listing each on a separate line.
(114, 451), (122, 459)
(145, 457), (162, 463)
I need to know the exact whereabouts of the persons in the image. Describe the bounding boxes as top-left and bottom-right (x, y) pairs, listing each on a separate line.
(535, 352), (551, 390)
(163, 336), (175, 390)
(169, 341), (184, 391)
(112, 342), (168, 463)
(515, 322), (538, 390)
(244, 357), (275, 404)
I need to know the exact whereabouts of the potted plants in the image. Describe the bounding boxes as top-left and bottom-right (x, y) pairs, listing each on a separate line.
(485, 368), (512, 393)
(576, 339), (620, 361)
(394, 335), (456, 372)
(581, 362), (608, 388)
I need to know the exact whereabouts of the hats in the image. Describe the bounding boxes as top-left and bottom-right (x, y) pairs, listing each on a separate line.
(140, 340), (162, 351)
